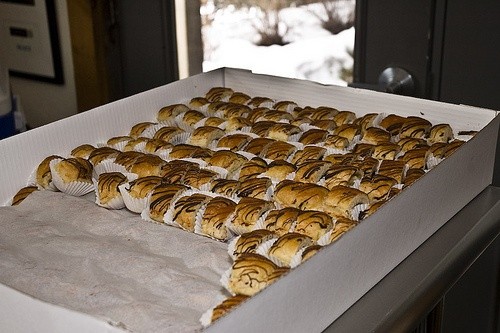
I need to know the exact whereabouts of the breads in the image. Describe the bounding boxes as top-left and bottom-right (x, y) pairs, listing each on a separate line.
(11, 86), (479, 323)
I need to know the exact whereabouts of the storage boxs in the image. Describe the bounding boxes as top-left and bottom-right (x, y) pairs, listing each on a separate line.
(0, 68), (499, 333)
